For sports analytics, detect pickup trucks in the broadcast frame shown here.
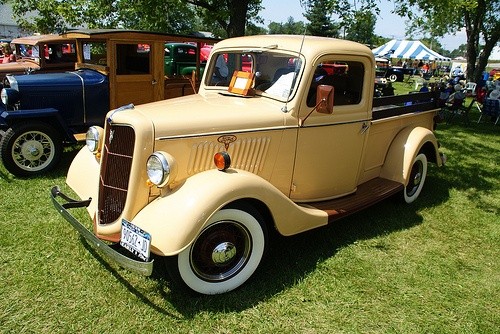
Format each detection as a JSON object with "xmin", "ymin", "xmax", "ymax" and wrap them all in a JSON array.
[
  {"xmin": 373, "ymin": 58, "xmax": 422, "ymax": 83},
  {"xmin": 51, "ymin": 32, "xmax": 445, "ymax": 291}
]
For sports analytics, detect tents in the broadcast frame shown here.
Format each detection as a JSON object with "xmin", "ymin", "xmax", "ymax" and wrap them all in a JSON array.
[
  {"xmin": 476, "ymin": 45, "xmax": 500, "ymax": 67},
  {"xmin": 371, "ymin": 40, "xmax": 451, "ymax": 64}
]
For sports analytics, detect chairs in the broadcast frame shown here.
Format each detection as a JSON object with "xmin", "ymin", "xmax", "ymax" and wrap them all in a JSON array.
[
  {"xmin": 61, "ymin": 53, "xmax": 76, "ymax": 63},
  {"xmin": 410, "ymin": 78, "xmax": 500, "ymax": 128},
  {"xmin": 320, "ymin": 74, "xmax": 349, "ymax": 108},
  {"xmin": 272, "ymin": 69, "xmax": 292, "ymax": 88}
]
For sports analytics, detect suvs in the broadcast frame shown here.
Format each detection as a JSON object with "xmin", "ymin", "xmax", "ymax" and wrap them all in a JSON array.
[
  {"xmin": 0, "ymin": 29, "xmax": 226, "ymax": 178},
  {"xmin": 1, "ymin": 33, "xmax": 93, "ymax": 85}
]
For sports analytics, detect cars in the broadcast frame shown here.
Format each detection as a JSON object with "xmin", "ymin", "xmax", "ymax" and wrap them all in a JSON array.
[{"xmin": 161, "ymin": 43, "xmax": 251, "ymax": 86}]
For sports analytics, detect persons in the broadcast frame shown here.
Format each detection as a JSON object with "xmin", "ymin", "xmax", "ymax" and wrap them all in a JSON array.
[
  {"xmin": 420, "ymin": 65, "xmax": 500, "ymax": 119},
  {"xmin": 1, "ymin": 42, "xmax": 16, "ymax": 64},
  {"xmin": 386, "ymin": 57, "xmax": 451, "ymax": 76},
  {"xmin": 307, "ymin": 66, "xmax": 329, "ymax": 106}
]
